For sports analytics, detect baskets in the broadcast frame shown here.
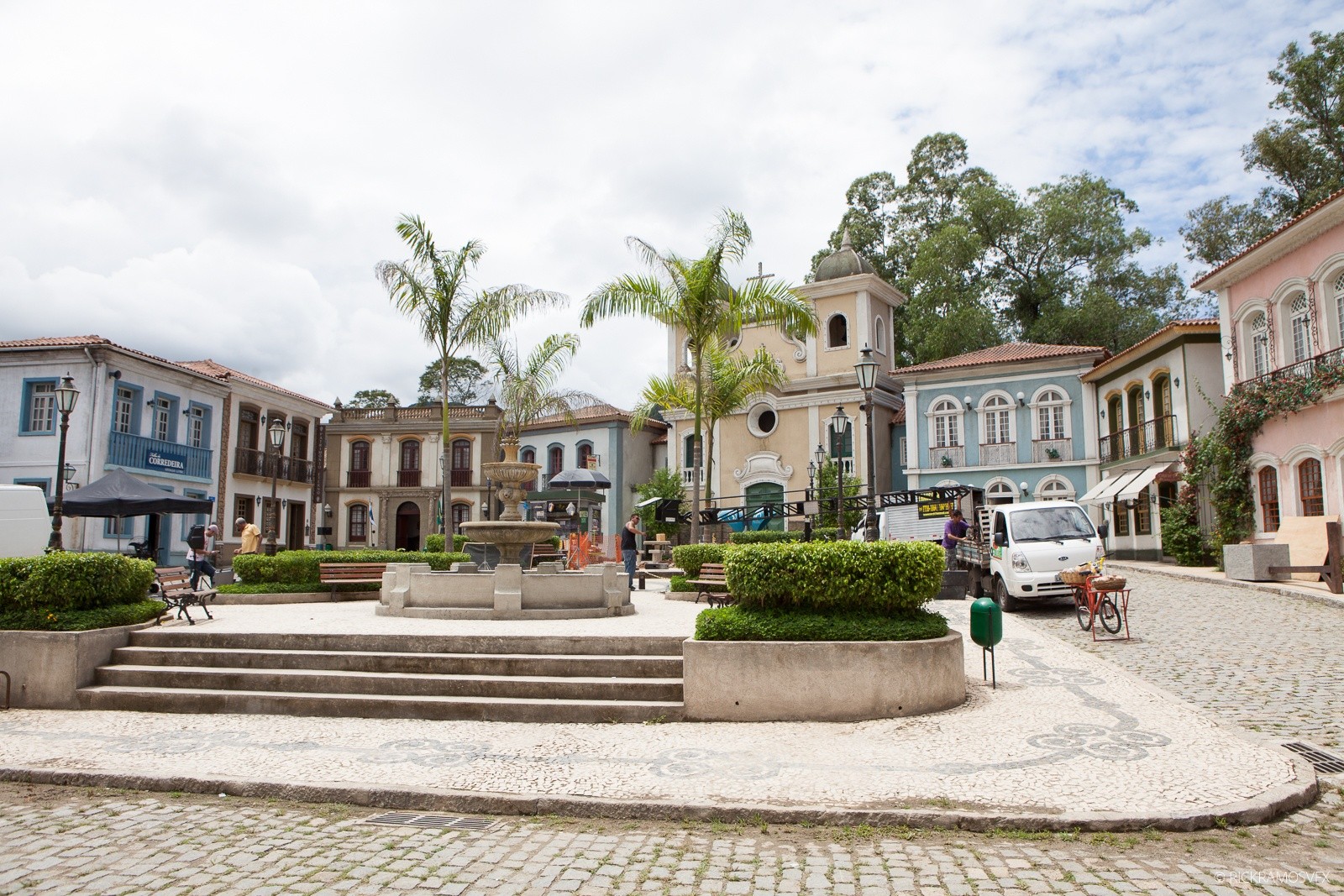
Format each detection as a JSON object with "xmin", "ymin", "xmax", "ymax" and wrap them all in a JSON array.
[
  {"xmin": 1058, "ymin": 571, "xmax": 1095, "ymax": 583},
  {"xmin": 1092, "ymin": 576, "xmax": 1127, "ymax": 590}
]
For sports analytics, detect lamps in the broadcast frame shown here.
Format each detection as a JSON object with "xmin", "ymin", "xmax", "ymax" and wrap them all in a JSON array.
[
  {"xmin": 480, "ymin": 501, "xmax": 489, "ymax": 517},
  {"xmin": 64, "ymin": 463, "xmax": 79, "ymax": 492},
  {"xmin": 1220, "ymin": 298, "xmax": 1315, "ymax": 361},
  {"xmin": 1174, "ymin": 377, "xmax": 1179, "ymax": 388},
  {"xmin": 282, "ymin": 499, "xmax": 287, "ymax": 509},
  {"xmin": 257, "ymin": 495, "xmax": 261, "ymax": 507},
  {"xmin": 1100, "ymin": 410, "xmax": 1105, "ymax": 418},
  {"xmin": 1105, "ymin": 502, "xmax": 1110, "ymax": 510},
  {"xmin": 1019, "ymin": 482, "xmax": 1028, "ymax": 497},
  {"xmin": 147, "ymin": 398, "xmax": 191, "ymax": 417},
  {"xmin": 286, "ymin": 422, "xmax": 291, "ymax": 432},
  {"xmin": 324, "ymin": 503, "xmax": 332, "ymax": 518},
  {"xmin": 1150, "ymin": 494, "xmax": 1157, "ymax": 504},
  {"xmin": 1017, "ymin": 392, "xmax": 1025, "ymax": 407},
  {"xmin": 964, "ymin": 396, "xmax": 972, "ymax": 411},
  {"xmin": 261, "ymin": 415, "xmax": 266, "ymax": 427},
  {"xmin": 1144, "ymin": 390, "xmax": 1149, "ymax": 400}
]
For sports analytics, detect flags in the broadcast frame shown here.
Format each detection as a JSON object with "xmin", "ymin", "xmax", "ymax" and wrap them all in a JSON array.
[
  {"xmin": 369, "ymin": 503, "xmax": 375, "ymax": 526},
  {"xmin": 437, "ymin": 500, "xmax": 442, "ymax": 526}
]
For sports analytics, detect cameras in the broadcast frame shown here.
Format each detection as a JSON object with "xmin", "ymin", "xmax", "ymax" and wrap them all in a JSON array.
[{"xmin": 212, "ymin": 550, "xmax": 220, "ymax": 555}]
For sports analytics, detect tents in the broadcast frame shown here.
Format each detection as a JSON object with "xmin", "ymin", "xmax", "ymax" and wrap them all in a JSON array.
[{"xmin": 45, "ymin": 467, "xmax": 213, "ymax": 555}]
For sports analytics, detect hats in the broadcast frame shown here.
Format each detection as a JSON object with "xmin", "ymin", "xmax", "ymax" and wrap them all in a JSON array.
[{"xmin": 209, "ymin": 524, "xmax": 219, "ymax": 535}]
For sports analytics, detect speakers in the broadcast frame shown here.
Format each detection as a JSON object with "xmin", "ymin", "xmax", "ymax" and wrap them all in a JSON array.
[{"xmin": 186, "ymin": 524, "xmax": 205, "ymax": 549}]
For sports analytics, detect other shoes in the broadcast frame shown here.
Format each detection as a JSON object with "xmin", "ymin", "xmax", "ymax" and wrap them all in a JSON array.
[{"xmin": 630, "ymin": 586, "xmax": 635, "ymax": 591}]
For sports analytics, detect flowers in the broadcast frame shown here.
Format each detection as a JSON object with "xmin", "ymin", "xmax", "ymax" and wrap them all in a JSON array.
[
  {"xmin": 940, "ymin": 455, "xmax": 952, "ymax": 465},
  {"xmin": 1045, "ymin": 449, "xmax": 1060, "ymax": 459}
]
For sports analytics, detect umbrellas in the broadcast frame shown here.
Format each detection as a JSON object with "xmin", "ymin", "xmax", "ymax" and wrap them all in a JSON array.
[{"xmin": 548, "ymin": 466, "xmax": 612, "ymax": 568}]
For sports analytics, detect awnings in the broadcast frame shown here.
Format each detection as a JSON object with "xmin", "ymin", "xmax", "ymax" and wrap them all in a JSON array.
[
  {"xmin": 1077, "ymin": 471, "xmax": 1128, "ymax": 506},
  {"xmin": 1118, "ymin": 463, "xmax": 1173, "ymax": 501},
  {"xmin": 1094, "ymin": 470, "xmax": 1144, "ymax": 504}
]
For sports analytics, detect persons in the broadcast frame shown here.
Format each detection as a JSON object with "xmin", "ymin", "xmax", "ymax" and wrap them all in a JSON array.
[
  {"xmin": 620, "ymin": 513, "xmax": 647, "ymax": 591},
  {"xmin": 942, "ymin": 510, "xmax": 976, "ymax": 570},
  {"xmin": 233, "ymin": 518, "xmax": 263, "ymax": 555},
  {"xmin": 186, "ymin": 525, "xmax": 218, "ymax": 592}
]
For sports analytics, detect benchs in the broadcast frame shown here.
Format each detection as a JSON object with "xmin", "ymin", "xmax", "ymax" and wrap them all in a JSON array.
[
  {"xmin": 532, "ymin": 544, "xmax": 569, "ymax": 571},
  {"xmin": 154, "ymin": 565, "xmax": 220, "ymax": 625},
  {"xmin": 685, "ymin": 563, "xmax": 737, "ymax": 608},
  {"xmin": 319, "ymin": 562, "xmax": 386, "ymax": 602}
]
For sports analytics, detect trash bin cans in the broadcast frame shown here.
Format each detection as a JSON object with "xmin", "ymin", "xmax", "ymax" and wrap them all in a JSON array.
[
  {"xmin": 326, "ymin": 543, "xmax": 332, "ymax": 551},
  {"xmin": 970, "ymin": 596, "xmax": 1003, "ymax": 648},
  {"xmin": 316, "ymin": 544, "xmax": 323, "ymax": 551}
]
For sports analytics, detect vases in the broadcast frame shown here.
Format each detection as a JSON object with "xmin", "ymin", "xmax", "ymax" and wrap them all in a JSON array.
[
  {"xmin": 941, "ymin": 464, "xmax": 953, "ymax": 468},
  {"xmin": 1049, "ymin": 458, "xmax": 1062, "ymax": 461}
]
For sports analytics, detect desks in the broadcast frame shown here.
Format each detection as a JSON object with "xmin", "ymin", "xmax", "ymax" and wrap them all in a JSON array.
[{"xmin": 643, "ymin": 540, "xmax": 671, "ymax": 562}]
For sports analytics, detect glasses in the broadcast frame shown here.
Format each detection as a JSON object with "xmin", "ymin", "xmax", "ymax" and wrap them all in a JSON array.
[{"xmin": 636, "ymin": 517, "xmax": 640, "ymax": 520}]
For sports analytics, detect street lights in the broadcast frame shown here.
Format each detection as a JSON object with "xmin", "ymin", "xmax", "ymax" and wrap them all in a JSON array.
[
  {"xmin": 482, "ymin": 476, "xmax": 493, "ymax": 520},
  {"xmin": 805, "ymin": 461, "xmax": 817, "ymax": 529},
  {"xmin": 854, "ymin": 342, "xmax": 882, "ymax": 544},
  {"xmin": 264, "ymin": 418, "xmax": 286, "ymax": 557},
  {"xmin": 814, "ymin": 443, "xmax": 826, "ymax": 526},
  {"xmin": 832, "ymin": 405, "xmax": 849, "ymax": 539},
  {"xmin": 439, "ymin": 452, "xmax": 446, "ymax": 535},
  {"xmin": 43, "ymin": 369, "xmax": 80, "ymax": 557}
]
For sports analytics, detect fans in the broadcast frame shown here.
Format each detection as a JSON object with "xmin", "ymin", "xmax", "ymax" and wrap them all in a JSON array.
[{"xmin": 126, "ymin": 536, "xmax": 151, "ymax": 559}]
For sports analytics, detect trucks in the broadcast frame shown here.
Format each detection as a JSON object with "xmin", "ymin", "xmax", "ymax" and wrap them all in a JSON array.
[
  {"xmin": 0, "ymin": 484, "xmax": 53, "ymax": 560},
  {"xmin": 956, "ymin": 501, "xmax": 1111, "ymax": 611},
  {"xmin": 851, "ymin": 484, "xmax": 986, "ymax": 549}
]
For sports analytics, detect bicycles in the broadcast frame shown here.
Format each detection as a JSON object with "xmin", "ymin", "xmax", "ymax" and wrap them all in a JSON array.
[{"xmin": 1073, "ymin": 552, "xmax": 1123, "ymax": 634}]
